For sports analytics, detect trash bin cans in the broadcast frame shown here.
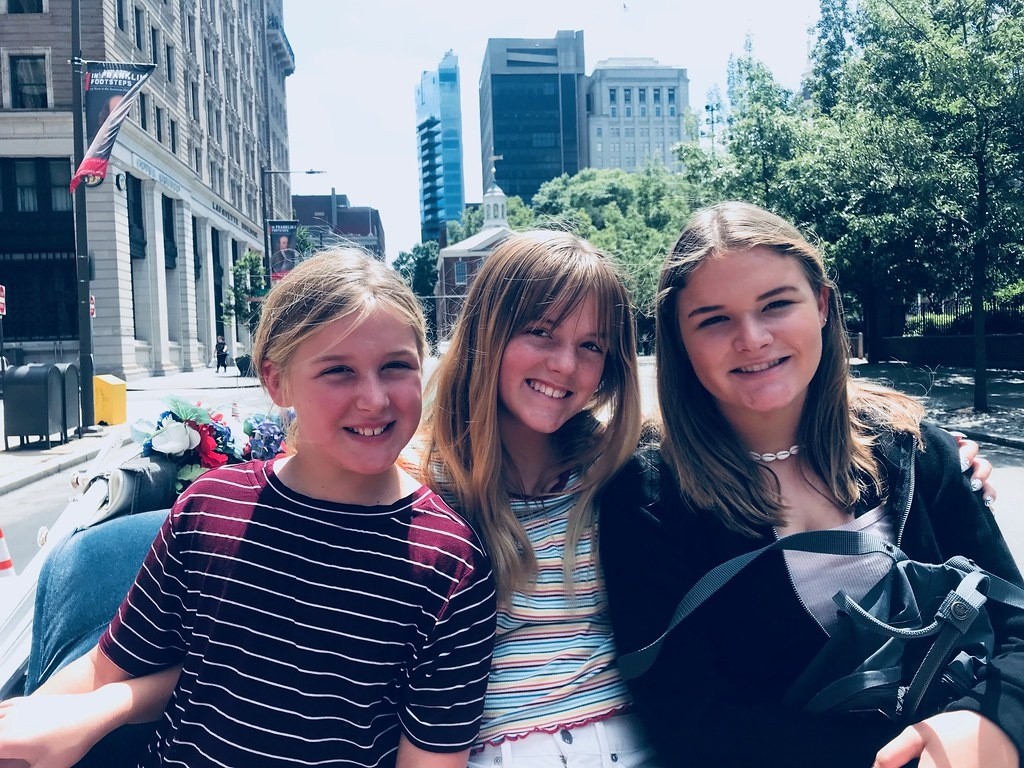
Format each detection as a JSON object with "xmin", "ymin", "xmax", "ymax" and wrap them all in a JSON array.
[
  {"xmin": 2, "ymin": 365, "xmax": 64, "ymax": 452},
  {"xmin": 643, "ymin": 341, "xmax": 655, "ymax": 356},
  {"xmin": 26, "ymin": 362, "xmax": 82, "ymax": 443},
  {"xmin": 636, "ymin": 341, "xmax": 643, "ymax": 356}
]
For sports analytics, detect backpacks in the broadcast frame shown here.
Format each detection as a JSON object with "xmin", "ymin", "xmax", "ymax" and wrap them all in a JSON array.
[{"xmin": 783, "ymin": 555, "xmax": 1024, "ymax": 768}]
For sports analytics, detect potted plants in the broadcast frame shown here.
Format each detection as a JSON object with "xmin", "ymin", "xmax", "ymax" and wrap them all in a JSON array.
[{"xmin": 219, "ymin": 248, "xmax": 270, "ymax": 379}]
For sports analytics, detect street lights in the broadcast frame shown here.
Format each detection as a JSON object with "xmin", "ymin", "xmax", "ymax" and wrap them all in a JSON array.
[
  {"xmin": 260, "ymin": 166, "xmax": 325, "ymax": 290},
  {"xmin": 699, "ymin": 102, "xmax": 722, "ymax": 162}
]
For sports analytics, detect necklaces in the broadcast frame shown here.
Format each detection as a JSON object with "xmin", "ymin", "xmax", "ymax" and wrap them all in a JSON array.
[{"xmin": 748, "ymin": 445, "xmax": 804, "ymax": 463}]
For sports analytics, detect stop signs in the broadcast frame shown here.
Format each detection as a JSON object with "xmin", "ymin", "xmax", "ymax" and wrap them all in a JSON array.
[
  {"xmin": 0, "ymin": 285, "xmax": 6, "ymax": 315},
  {"xmin": 89, "ymin": 295, "xmax": 96, "ymax": 317}
]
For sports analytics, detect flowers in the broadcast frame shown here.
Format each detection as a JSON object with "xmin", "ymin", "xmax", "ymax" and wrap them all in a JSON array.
[{"xmin": 144, "ymin": 392, "xmax": 295, "ymax": 500}]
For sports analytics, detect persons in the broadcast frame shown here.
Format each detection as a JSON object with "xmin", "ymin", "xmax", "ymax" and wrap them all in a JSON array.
[
  {"xmin": 0, "ymin": 228, "xmax": 656, "ymax": 768},
  {"xmin": 272, "ymin": 236, "xmax": 295, "ymax": 273},
  {"xmin": 598, "ymin": 201, "xmax": 1024, "ymax": 768},
  {"xmin": 213, "ymin": 335, "xmax": 228, "ymax": 373},
  {"xmin": 0, "ymin": 247, "xmax": 499, "ymax": 768}
]
